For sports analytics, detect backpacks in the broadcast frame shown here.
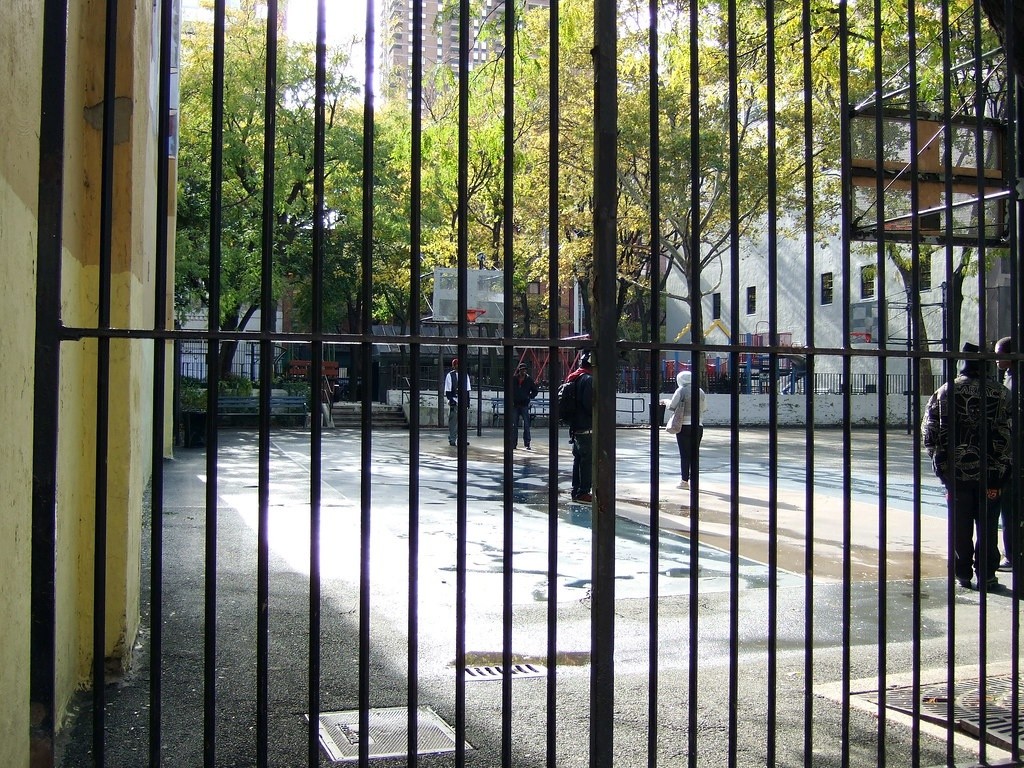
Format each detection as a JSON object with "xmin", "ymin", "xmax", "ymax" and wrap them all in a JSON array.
[{"xmin": 557, "ymin": 371, "xmax": 585, "ymax": 423}]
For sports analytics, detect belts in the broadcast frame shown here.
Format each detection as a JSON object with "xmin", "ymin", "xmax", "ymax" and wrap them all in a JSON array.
[{"xmin": 573, "ymin": 430, "xmax": 592, "ymax": 434}]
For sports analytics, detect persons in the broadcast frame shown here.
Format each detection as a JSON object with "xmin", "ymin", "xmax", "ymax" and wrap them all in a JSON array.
[
  {"xmin": 659, "ymin": 371, "xmax": 706, "ymax": 490},
  {"xmin": 512, "ymin": 363, "xmax": 538, "ymax": 451},
  {"xmin": 445, "ymin": 359, "xmax": 471, "ymax": 446},
  {"xmin": 920, "ymin": 342, "xmax": 1012, "ymax": 593},
  {"xmin": 990, "ymin": 337, "xmax": 1012, "ymax": 571},
  {"xmin": 564, "ymin": 354, "xmax": 593, "ymax": 504}
]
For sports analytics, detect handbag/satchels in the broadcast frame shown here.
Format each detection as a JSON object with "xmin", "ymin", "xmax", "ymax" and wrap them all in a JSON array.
[{"xmin": 665, "ymin": 388, "xmax": 685, "ymax": 433}]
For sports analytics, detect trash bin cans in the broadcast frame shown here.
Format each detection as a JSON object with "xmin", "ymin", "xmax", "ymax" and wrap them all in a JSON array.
[{"xmin": 649, "ymin": 403, "xmax": 666, "ymax": 426}]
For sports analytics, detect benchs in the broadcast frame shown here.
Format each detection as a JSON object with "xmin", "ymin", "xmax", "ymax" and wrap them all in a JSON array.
[
  {"xmin": 215, "ymin": 395, "xmax": 309, "ymax": 430},
  {"xmin": 490, "ymin": 398, "xmax": 549, "ymax": 428}
]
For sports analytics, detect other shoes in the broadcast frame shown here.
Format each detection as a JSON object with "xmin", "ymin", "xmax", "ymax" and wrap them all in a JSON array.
[
  {"xmin": 450, "ymin": 441, "xmax": 456, "ymax": 446},
  {"xmin": 977, "ymin": 575, "xmax": 998, "ymax": 589},
  {"xmin": 571, "ymin": 493, "xmax": 578, "ymax": 501},
  {"xmin": 955, "ymin": 572, "xmax": 972, "ymax": 586},
  {"xmin": 467, "ymin": 442, "xmax": 469, "ymax": 445},
  {"xmin": 577, "ymin": 493, "xmax": 593, "ymax": 503},
  {"xmin": 513, "ymin": 445, "xmax": 516, "ymax": 449},
  {"xmin": 999, "ymin": 556, "xmax": 1013, "ymax": 572},
  {"xmin": 526, "ymin": 446, "xmax": 531, "ymax": 450}
]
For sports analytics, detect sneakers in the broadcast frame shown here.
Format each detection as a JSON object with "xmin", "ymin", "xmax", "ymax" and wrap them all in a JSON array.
[{"xmin": 677, "ymin": 481, "xmax": 689, "ymax": 489}]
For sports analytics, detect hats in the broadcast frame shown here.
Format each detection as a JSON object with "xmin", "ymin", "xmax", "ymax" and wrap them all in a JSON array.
[
  {"xmin": 579, "ymin": 351, "xmax": 591, "ymax": 363},
  {"xmin": 518, "ymin": 363, "xmax": 528, "ymax": 370},
  {"xmin": 452, "ymin": 359, "xmax": 458, "ymax": 366}
]
